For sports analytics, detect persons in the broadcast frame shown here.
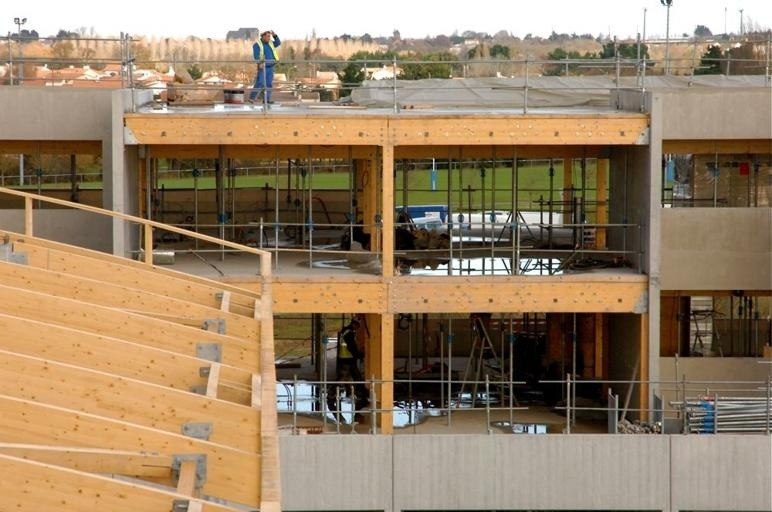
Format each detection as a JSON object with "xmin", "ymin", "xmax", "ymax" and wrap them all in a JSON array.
[
  {"xmin": 335, "ymin": 316, "xmax": 370, "ymax": 400},
  {"xmin": 247, "ymin": 30, "xmax": 281, "ymax": 105}
]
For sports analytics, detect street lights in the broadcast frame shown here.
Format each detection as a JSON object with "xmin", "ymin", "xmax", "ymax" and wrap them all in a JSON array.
[
  {"xmin": 659, "ymin": 0, "xmax": 673, "ymax": 78},
  {"xmin": 13, "ymin": 17, "xmax": 29, "ymax": 187}
]
